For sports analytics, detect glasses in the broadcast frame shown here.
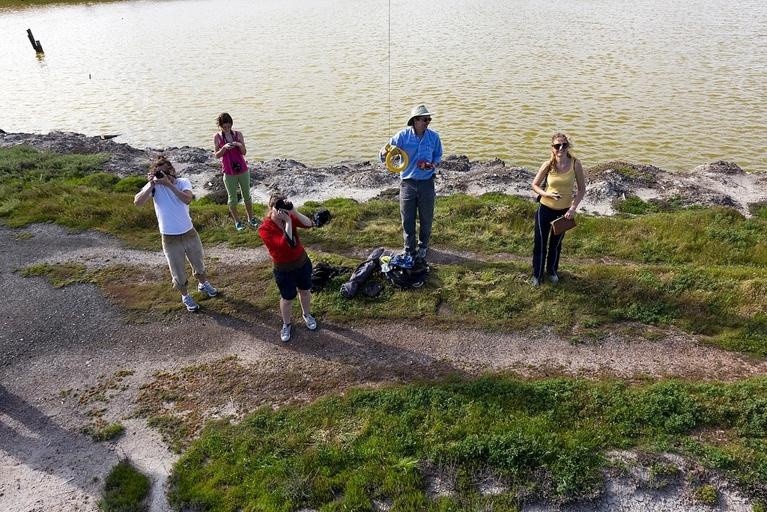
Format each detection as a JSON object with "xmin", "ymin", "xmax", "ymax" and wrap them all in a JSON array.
[
  {"xmin": 551, "ymin": 143, "xmax": 570, "ymax": 150},
  {"xmin": 422, "ymin": 118, "xmax": 432, "ymax": 122}
]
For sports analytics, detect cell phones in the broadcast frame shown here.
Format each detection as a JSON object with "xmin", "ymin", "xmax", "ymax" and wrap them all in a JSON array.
[
  {"xmin": 229, "ymin": 143, "xmax": 234, "ymax": 147},
  {"xmin": 554, "ymin": 193, "xmax": 561, "ymax": 200}
]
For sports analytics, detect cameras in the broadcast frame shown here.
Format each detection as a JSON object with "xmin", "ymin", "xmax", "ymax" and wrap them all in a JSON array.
[
  {"xmin": 232, "ymin": 162, "xmax": 243, "ymax": 173},
  {"xmin": 153, "ymin": 167, "xmax": 170, "ymax": 181},
  {"xmin": 274, "ymin": 198, "xmax": 294, "ymax": 217}
]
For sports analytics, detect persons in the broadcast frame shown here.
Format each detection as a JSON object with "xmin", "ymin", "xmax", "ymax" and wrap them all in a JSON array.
[
  {"xmin": 213, "ymin": 110, "xmax": 257, "ymax": 231},
  {"xmin": 530, "ymin": 132, "xmax": 586, "ymax": 283},
  {"xmin": 378, "ymin": 103, "xmax": 445, "ymax": 264},
  {"xmin": 133, "ymin": 157, "xmax": 218, "ymax": 312},
  {"xmin": 256, "ymin": 185, "xmax": 320, "ymax": 342}
]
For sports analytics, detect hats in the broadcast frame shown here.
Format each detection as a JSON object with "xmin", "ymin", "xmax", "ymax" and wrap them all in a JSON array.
[{"xmin": 407, "ymin": 104, "xmax": 435, "ymax": 126}]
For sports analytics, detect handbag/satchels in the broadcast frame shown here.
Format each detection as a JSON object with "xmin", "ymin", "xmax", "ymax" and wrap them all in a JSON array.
[
  {"xmin": 315, "ymin": 210, "xmax": 331, "ymax": 227},
  {"xmin": 531, "ymin": 176, "xmax": 547, "ymax": 203},
  {"xmin": 310, "ymin": 261, "xmax": 348, "ymax": 291}
]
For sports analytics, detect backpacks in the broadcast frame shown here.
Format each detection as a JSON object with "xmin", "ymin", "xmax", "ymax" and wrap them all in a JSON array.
[{"xmin": 384, "ymin": 254, "xmax": 432, "ymax": 289}]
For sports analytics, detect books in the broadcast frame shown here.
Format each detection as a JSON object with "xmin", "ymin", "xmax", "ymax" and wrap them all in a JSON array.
[{"xmin": 550, "ymin": 215, "xmax": 576, "ymax": 235}]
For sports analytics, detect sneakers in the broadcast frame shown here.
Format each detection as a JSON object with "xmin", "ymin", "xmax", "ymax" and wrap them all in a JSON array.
[
  {"xmin": 417, "ymin": 247, "xmax": 429, "ymax": 258},
  {"xmin": 280, "ymin": 323, "xmax": 292, "ymax": 342},
  {"xmin": 198, "ymin": 281, "xmax": 219, "ymax": 297},
  {"xmin": 182, "ymin": 293, "xmax": 200, "ymax": 312},
  {"xmin": 533, "ymin": 276, "xmax": 541, "ymax": 286},
  {"xmin": 248, "ymin": 217, "xmax": 263, "ymax": 228},
  {"xmin": 302, "ymin": 314, "xmax": 317, "ymax": 329},
  {"xmin": 234, "ymin": 221, "xmax": 246, "ymax": 231},
  {"xmin": 549, "ymin": 275, "xmax": 558, "ymax": 283}
]
